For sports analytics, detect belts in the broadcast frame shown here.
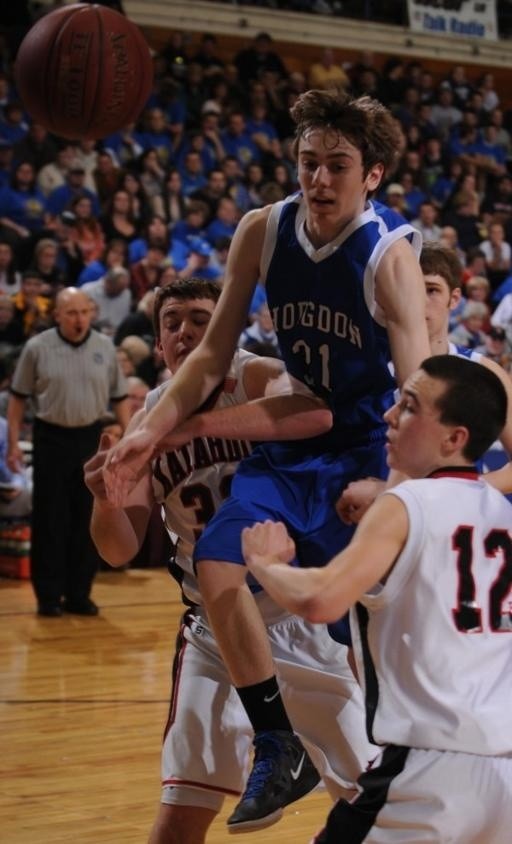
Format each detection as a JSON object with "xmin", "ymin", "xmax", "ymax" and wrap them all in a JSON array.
[{"xmin": 35, "ymin": 417, "xmax": 104, "ymax": 435}]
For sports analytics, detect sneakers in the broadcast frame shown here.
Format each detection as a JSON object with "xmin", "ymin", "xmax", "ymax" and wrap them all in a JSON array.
[{"xmin": 226, "ymin": 729, "xmax": 325, "ymax": 838}]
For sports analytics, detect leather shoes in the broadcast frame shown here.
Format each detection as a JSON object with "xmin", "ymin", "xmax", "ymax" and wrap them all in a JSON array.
[
  {"xmin": 36, "ymin": 598, "xmax": 65, "ymax": 618},
  {"xmin": 63, "ymin": 594, "xmax": 99, "ymax": 617}
]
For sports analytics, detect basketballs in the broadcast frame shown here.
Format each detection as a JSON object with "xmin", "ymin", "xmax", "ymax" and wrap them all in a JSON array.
[{"xmin": 14, "ymin": 5, "xmax": 152, "ymax": 135}]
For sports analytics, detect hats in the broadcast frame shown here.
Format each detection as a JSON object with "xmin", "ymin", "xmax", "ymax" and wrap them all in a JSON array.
[
  {"xmin": 189, "ymin": 238, "xmax": 212, "ymax": 258},
  {"xmin": 30, "ymin": 231, "xmax": 61, "ymax": 255}
]
